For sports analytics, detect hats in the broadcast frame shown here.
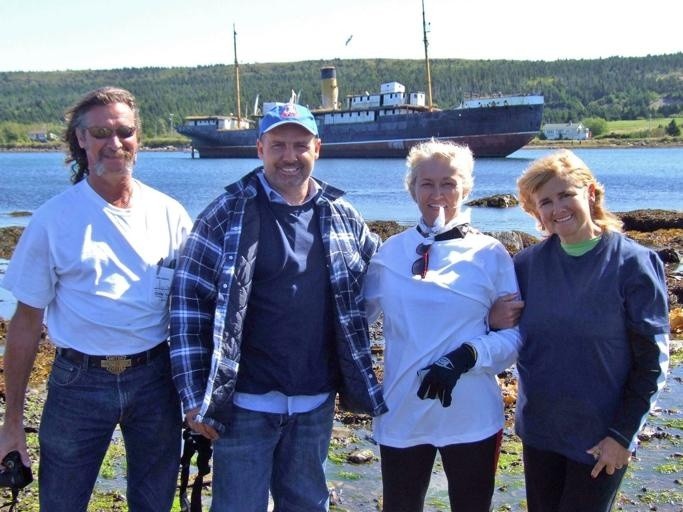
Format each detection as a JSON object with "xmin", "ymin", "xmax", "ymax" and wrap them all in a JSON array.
[{"xmin": 257, "ymin": 103, "xmax": 319, "ymax": 140}]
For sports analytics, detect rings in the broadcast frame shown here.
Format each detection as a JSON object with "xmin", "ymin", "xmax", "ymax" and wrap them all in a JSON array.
[{"xmin": 616, "ymin": 466, "xmax": 623, "ymax": 470}]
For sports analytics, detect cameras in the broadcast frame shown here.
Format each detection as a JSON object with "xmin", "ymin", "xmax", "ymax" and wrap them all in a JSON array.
[{"xmin": 0, "ymin": 451, "xmax": 33, "ymax": 489}]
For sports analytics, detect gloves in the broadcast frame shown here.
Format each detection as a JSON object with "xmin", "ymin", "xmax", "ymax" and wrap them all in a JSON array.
[{"xmin": 417, "ymin": 343, "xmax": 476, "ymax": 408}]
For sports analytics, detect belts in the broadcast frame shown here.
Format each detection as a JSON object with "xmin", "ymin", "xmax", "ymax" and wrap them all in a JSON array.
[{"xmin": 55, "ymin": 338, "xmax": 168, "ymax": 376}]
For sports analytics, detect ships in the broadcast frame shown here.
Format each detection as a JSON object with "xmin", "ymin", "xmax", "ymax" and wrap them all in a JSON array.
[{"xmin": 171, "ymin": 0, "xmax": 547, "ymax": 161}]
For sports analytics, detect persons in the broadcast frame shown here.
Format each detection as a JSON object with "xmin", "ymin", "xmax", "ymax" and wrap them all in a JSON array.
[
  {"xmin": 0, "ymin": 87, "xmax": 194, "ymax": 512},
  {"xmin": 169, "ymin": 104, "xmax": 389, "ymax": 512},
  {"xmin": 489, "ymin": 149, "xmax": 670, "ymax": 512},
  {"xmin": 364, "ymin": 136, "xmax": 523, "ymax": 512}
]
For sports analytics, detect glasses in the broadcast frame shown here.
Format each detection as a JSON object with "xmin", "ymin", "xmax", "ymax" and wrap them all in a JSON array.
[
  {"xmin": 85, "ymin": 127, "xmax": 138, "ymax": 138},
  {"xmin": 412, "ymin": 240, "xmax": 433, "ymax": 279}
]
